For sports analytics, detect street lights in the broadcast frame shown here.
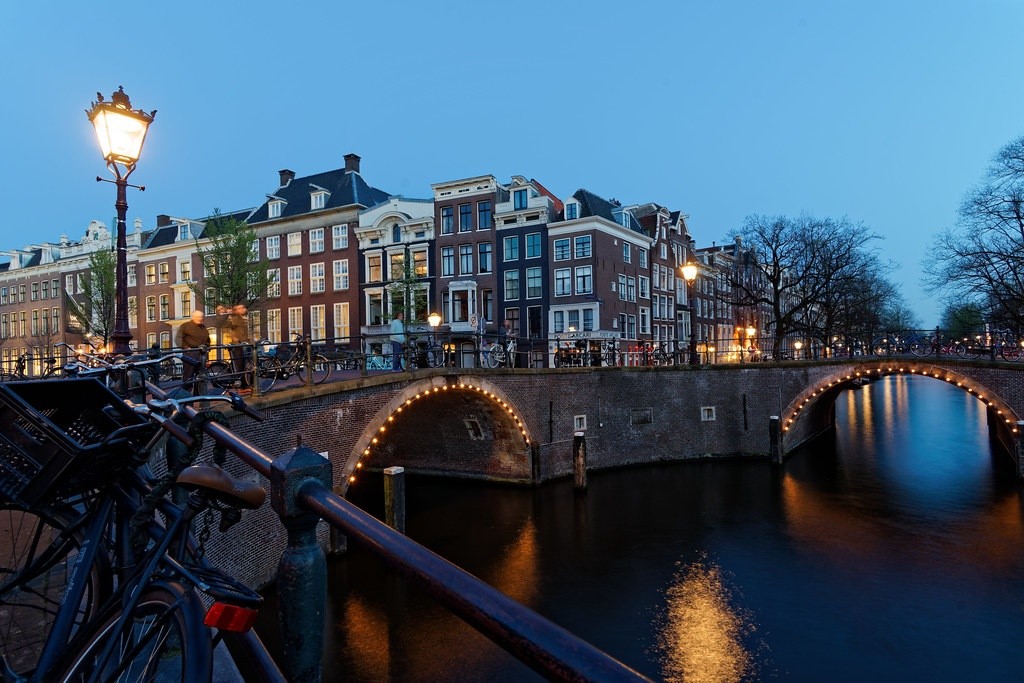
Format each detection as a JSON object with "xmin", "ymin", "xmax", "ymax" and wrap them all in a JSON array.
[
  {"xmin": 746, "ymin": 326, "xmax": 756, "ymax": 349},
  {"xmin": 84, "ymin": 84, "xmax": 158, "ymax": 356},
  {"xmin": 794, "ymin": 341, "xmax": 803, "ymax": 360},
  {"xmin": 679, "ymin": 260, "xmax": 701, "ymax": 366}
]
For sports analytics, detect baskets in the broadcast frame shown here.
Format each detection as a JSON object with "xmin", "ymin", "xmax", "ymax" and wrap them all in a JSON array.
[{"xmin": 0, "ymin": 377, "xmax": 151, "ymax": 502}]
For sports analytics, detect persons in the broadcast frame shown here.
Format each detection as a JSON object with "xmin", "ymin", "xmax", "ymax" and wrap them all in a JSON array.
[
  {"xmin": 498, "ymin": 320, "xmax": 512, "ymax": 367},
  {"xmin": 390, "ymin": 312, "xmax": 406, "ymax": 372},
  {"xmin": 174, "ymin": 311, "xmax": 211, "ymax": 396}
]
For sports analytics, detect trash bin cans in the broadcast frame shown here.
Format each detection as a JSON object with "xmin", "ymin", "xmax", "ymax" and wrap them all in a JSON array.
[{"xmin": 224, "ymin": 341, "xmax": 290, "ymax": 388}]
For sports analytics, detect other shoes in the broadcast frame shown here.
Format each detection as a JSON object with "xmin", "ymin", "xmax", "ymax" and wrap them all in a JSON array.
[
  {"xmin": 392, "ymin": 369, "xmax": 402, "ymax": 372},
  {"xmin": 239, "ymin": 389, "xmax": 253, "ymax": 395}
]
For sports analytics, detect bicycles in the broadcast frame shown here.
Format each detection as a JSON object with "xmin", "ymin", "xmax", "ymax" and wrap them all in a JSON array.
[
  {"xmin": 487, "ymin": 332, "xmax": 518, "ymax": 369},
  {"xmin": 554, "ymin": 339, "xmax": 623, "ymax": 368},
  {"xmin": 650, "ymin": 339, "xmax": 689, "ymax": 367},
  {"xmin": 956, "ymin": 328, "xmax": 1023, "ymax": 363},
  {"xmin": 871, "ymin": 327, "xmax": 934, "ymax": 358},
  {"xmin": 398, "ymin": 325, "xmax": 447, "ymax": 373},
  {"xmin": 0, "ymin": 333, "xmax": 396, "ymax": 683}
]
